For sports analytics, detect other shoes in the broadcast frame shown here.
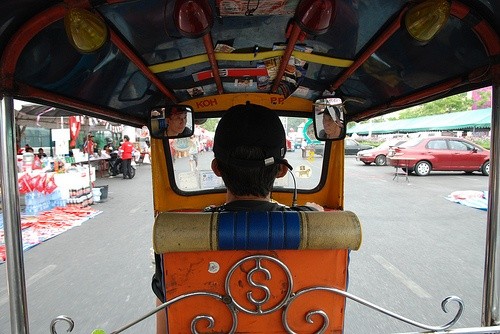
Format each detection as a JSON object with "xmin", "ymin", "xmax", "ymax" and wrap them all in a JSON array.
[{"xmin": 109, "ymin": 175, "xmax": 116, "ymax": 178}]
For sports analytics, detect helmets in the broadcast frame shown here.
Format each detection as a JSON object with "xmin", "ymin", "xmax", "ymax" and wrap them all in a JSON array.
[{"xmin": 106, "ymin": 137, "xmax": 113, "ymax": 143}]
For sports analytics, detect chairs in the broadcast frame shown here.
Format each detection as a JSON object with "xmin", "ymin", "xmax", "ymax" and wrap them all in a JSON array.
[{"xmin": 153, "ymin": 209, "xmax": 362, "ymax": 334}]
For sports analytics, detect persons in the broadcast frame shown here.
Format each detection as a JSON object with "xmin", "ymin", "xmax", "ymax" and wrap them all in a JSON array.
[
  {"xmin": 16, "ymin": 135, "xmax": 137, "ymax": 180},
  {"xmin": 318, "ymin": 105, "xmax": 345, "ymax": 139},
  {"xmin": 152, "ymin": 105, "xmax": 192, "ymax": 138},
  {"xmin": 150, "ymin": 101, "xmax": 320, "ymax": 304}
]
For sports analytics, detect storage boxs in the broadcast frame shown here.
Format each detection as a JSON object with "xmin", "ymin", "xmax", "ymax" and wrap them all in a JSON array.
[{"xmin": 95, "ymin": 185, "xmax": 109, "ymax": 200}]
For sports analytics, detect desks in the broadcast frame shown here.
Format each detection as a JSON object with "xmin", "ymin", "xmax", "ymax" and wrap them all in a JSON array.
[
  {"xmin": 76, "ymin": 157, "xmax": 111, "ymax": 180},
  {"xmin": 389, "ymin": 157, "xmax": 416, "ymax": 181}
]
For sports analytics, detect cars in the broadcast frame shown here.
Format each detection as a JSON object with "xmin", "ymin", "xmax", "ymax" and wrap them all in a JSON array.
[
  {"xmin": 386, "ymin": 136, "xmax": 490, "ymax": 177},
  {"xmin": 357, "ymin": 139, "xmax": 407, "ymax": 166},
  {"xmin": 311, "ymin": 138, "xmax": 376, "ymax": 155}
]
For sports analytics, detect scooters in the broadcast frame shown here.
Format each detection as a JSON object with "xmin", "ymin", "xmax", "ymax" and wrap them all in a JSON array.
[{"xmin": 107, "ymin": 159, "xmax": 136, "ymax": 179}]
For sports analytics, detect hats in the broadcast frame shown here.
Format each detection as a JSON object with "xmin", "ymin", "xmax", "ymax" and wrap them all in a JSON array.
[
  {"xmin": 165, "ymin": 107, "xmax": 191, "ymax": 117},
  {"xmin": 317, "ymin": 105, "xmax": 340, "ymax": 119},
  {"xmin": 213, "ymin": 101, "xmax": 294, "ymax": 170}
]
